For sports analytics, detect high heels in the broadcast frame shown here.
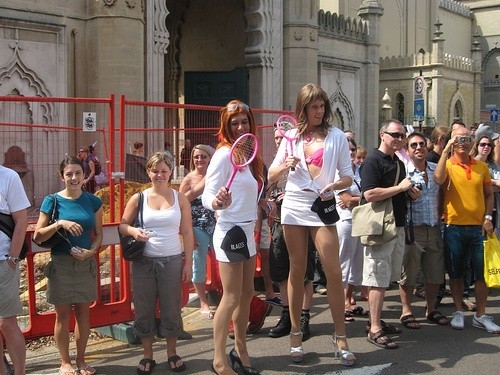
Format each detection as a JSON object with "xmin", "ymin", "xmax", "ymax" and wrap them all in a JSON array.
[
  {"xmin": 210, "ymin": 359, "xmax": 241, "ymax": 375},
  {"xmin": 229, "ymin": 347, "xmax": 260, "ymax": 375},
  {"xmin": 289, "ymin": 329, "xmax": 304, "ymax": 363},
  {"xmin": 329, "ymin": 331, "xmax": 356, "ymax": 366}
]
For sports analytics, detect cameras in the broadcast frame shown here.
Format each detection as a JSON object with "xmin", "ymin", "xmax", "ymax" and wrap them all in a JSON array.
[
  {"xmin": 406, "ymin": 183, "xmax": 423, "ymax": 201},
  {"xmin": 457, "ymin": 136, "xmax": 472, "ymax": 146},
  {"xmin": 142, "ymin": 230, "xmax": 158, "ymax": 237}
]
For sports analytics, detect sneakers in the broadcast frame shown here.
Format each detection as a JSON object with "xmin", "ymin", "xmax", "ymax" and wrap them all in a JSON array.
[
  {"xmin": 472, "ymin": 312, "xmax": 500, "ymax": 334},
  {"xmin": 450, "ymin": 311, "xmax": 465, "ymax": 330},
  {"xmin": 462, "ymin": 296, "xmax": 476, "ymax": 311},
  {"xmin": 416, "ymin": 287, "xmax": 425, "ymax": 297},
  {"xmin": 316, "ymin": 284, "xmax": 325, "ymax": 294},
  {"xmin": 248, "ymin": 300, "xmax": 273, "ymax": 334}
]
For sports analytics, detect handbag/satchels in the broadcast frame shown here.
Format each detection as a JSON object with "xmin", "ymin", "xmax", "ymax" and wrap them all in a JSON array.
[
  {"xmin": 32, "ymin": 218, "xmax": 73, "ymax": 249},
  {"xmin": 122, "ymin": 223, "xmax": 146, "ymax": 260},
  {"xmin": 93, "ymin": 162, "xmax": 101, "ymax": 175},
  {"xmin": 482, "ymin": 233, "xmax": 500, "ymax": 289},
  {"xmin": 0, "ymin": 212, "xmax": 28, "ymax": 259},
  {"xmin": 350, "ymin": 196, "xmax": 398, "ymax": 247}
]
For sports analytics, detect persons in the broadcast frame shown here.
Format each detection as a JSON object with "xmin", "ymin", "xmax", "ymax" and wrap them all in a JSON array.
[
  {"xmin": 164, "ymin": 141, "xmax": 177, "ymax": 170},
  {"xmin": 179, "ymin": 143, "xmax": 216, "ymax": 320},
  {"xmin": 268, "ymin": 122, "xmax": 316, "ymax": 342},
  {"xmin": 344, "ymin": 131, "xmax": 353, "ymax": 138},
  {"xmin": 396, "ymin": 133, "xmax": 449, "ymax": 329},
  {"xmin": 1, "ymin": 163, "xmax": 31, "ymax": 375},
  {"xmin": 425, "ymin": 132, "xmax": 479, "ymax": 311},
  {"xmin": 470, "ymin": 123, "xmax": 480, "ymax": 136},
  {"xmin": 466, "ymin": 135, "xmax": 500, "ymax": 286},
  {"xmin": 89, "ymin": 145, "xmax": 98, "ymax": 188},
  {"xmin": 312, "ymin": 251, "xmax": 327, "ymax": 296},
  {"xmin": 267, "ymin": 83, "xmax": 356, "ymax": 366},
  {"xmin": 468, "ymin": 125, "xmax": 500, "ymax": 168},
  {"xmin": 451, "ymin": 121, "xmax": 465, "ymax": 133},
  {"xmin": 76, "ymin": 146, "xmax": 95, "ymax": 193},
  {"xmin": 132, "ymin": 143, "xmax": 144, "ymax": 157},
  {"xmin": 434, "ymin": 127, "xmax": 500, "ymax": 335},
  {"xmin": 180, "ymin": 139, "xmax": 193, "ymax": 170},
  {"xmin": 359, "ymin": 119, "xmax": 422, "ymax": 349},
  {"xmin": 118, "ymin": 152, "xmax": 193, "ymax": 375},
  {"xmin": 416, "ymin": 126, "xmax": 451, "ymax": 297},
  {"xmin": 350, "ymin": 144, "xmax": 369, "ymax": 305},
  {"xmin": 258, "ymin": 162, "xmax": 282, "ymax": 306},
  {"xmin": 333, "ymin": 136, "xmax": 372, "ymax": 322},
  {"xmin": 33, "ymin": 156, "xmax": 104, "ymax": 375},
  {"xmin": 155, "ymin": 224, "xmax": 198, "ymax": 340},
  {"xmin": 207, "ymin": 208, "xmax": 273, "ymax": 339},
  {"xmin": 201, "ymin": 100, "xmax": 277, "ymax": 375}
]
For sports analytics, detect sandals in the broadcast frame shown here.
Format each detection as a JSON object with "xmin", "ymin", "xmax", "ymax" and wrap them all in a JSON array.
[
  {"xmin": 344, "ymin": 306, "xmax": 368, "ymax": 317},
  {"xmin": 367, "ymin": 326, "xmax": 398, "ymax": 349},
  {"xmin": 366, "ymin": 320, "xmax": 401, "ymax": 335},
  {"xmin": 200, "ymin": 309, "xmax": 213, "ymax": 320},
  {"xmin": 344, "ymin": 307, "xmax": 355, "ymax": 321}
]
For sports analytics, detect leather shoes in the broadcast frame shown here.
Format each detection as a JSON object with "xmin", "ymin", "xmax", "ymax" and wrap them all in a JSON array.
[
  {"xmin": 268, "ymin": 312, "xmax": 291, "ymax": 338},
  {"xmin": 300, "ymin": 314, "xmax": 311, "ymax": 342}
]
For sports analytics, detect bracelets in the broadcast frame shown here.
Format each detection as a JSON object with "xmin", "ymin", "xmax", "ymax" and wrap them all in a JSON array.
[{"xmin": 485, "ymin": 214, "xmax": 493, "ymax": 221}]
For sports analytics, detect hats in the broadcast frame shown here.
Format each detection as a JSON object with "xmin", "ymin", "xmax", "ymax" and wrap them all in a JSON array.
[
  {"xmin": 221, "ymin": 225, "xmax": 251, "ymax": 264},
  {"xmin": 310, "ymin": 195, "xmax": 340, "ymax": 224},
  {"xmin": 274, "ymin": 122, "xmax": 294, "ymax": 132},
  {"xmin": 474, "ymin": 125, "xmax": 499, "ymax": 140}
]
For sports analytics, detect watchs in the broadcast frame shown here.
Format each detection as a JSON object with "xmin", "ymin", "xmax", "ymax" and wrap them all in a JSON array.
[{"xmin": 7, "ymin": 256, "xmax": 19, "ymax": 264}]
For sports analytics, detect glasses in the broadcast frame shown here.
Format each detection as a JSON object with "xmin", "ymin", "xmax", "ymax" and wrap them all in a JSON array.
[
  {"xmin": 274, "ymin": 121, "xmax": 288, "ymax": 128},
  {"xmin": 350, "ymin": 147, "xmax": 356, "ymax": 150},
  {"xmin": 479, "ymin": 142, "xmax": 492, "ymax": 146},
  {"xmin": 79, "ymin": 151, "xmax": 85, "ymax": 153},
  {"xmin": 410, "ymin": 142, "xmax": 426, "ymax": 149},
  {"xmin": 383, "ymin": 131, "xmax": 406, "ymax": 140},
  {"xmin": 227, "ymin": 103, "xmax": 251, "ymax": 112},
  {"xmin": 192, "ymin": 154, "xmax": 209, "ymax": 159}
]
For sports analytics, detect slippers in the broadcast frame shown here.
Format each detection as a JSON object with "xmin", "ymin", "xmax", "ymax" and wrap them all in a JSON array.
[
  {"xmin": 424, "ymin": 310, "xmax": 449, "ymax": 326},
  {"xmin": 264, "ymin": 296, "xmax": 284, "ymax": 306},
  {"xmin": 168, "ymin": 355, "xmax": 185, "ymax": 372},
  {"xmin": 136, "ymin": 359, "xmax": 156, "ymax": 375},
  {"xmin": 72, "ymin": 365, "xmax": 96, "ymax": 375},
  {"xmin": 399, "ymin": 313, "xmax": 422, "ymax": 329},
  {"xmin": 59, "ymin": 367, "xmax": 76, "ymax": 375}
]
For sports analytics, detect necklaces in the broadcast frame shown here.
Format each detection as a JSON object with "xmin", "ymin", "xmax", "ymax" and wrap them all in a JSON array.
[
  {"xmin": 416, "ymin": 167, "xmax": 427, "ymax": 177},
  {"xmin": 305, "ymin": 128, "xmax": 314, "ymax": 142}
]
